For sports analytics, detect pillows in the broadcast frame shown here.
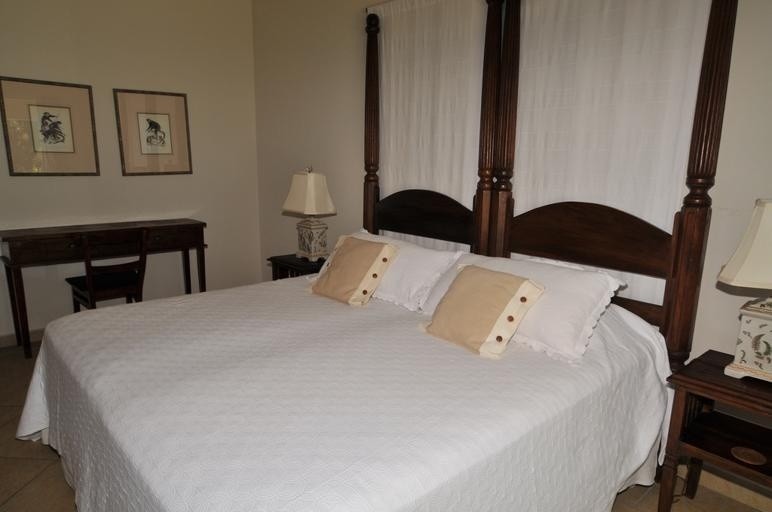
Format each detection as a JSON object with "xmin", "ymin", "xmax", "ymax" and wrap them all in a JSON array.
[
  {"xmin": 309, "ymin": 235, "xmax": 396, "ymax": 309},
  {"xmin": 424, "ymin": 264, "xmax": 544, "ymax": 358},
  {"xmin": 421, "ymin": 251, "xmax": 626, "ymax": 361},
  {"xmin": 348, "ymin": 230, "xmax": 463, "ymax": 311}
]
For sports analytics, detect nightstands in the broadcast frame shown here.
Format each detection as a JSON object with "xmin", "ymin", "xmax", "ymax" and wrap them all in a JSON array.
[
  {"xmin": 267, "ymin": 254, "xmax": 326, "ymax": 281},
  {"xmin": 658, "ymin": 350, "xmax": 772, "ymax": 512}
]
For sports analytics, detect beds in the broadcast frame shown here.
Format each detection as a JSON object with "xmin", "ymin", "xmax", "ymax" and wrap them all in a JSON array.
[{"xmin": 42, "ymin": 0, "xmax": 739, "ymax": 512}]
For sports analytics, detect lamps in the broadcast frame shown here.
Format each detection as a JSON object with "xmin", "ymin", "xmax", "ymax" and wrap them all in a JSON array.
[
  {"xmin": 282, "ymin": 164, "xmax": 337, "ymax": 261},
  {"xmin": 717, "ymin": 200, "xmax": 772, "ymax": 384}
]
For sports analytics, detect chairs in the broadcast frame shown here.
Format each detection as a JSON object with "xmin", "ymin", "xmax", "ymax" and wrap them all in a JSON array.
[{"xmin": 66, "ymin": 229, "xmax": 149, "ymax": 313}]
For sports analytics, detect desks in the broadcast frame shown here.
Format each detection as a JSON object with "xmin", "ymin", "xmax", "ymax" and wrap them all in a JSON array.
[{"xmin": 0, "ymin": 218, "xmax": 208, "ymax": 359}]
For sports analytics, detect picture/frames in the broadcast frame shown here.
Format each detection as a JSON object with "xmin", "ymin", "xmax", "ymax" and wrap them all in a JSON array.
[
  {"xmin": 113, "ymin": 87, "xmax": 194, "ymax": 176},
  {"xmin": 0, "ymin": 75, "xmax": 101, "ymax": 176}
]
